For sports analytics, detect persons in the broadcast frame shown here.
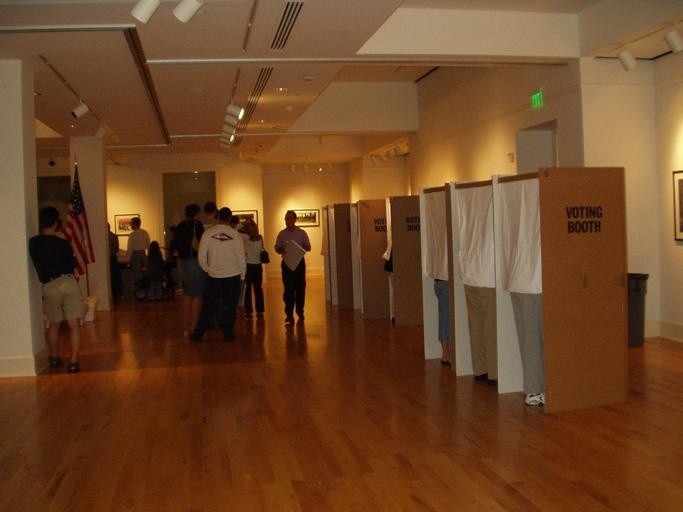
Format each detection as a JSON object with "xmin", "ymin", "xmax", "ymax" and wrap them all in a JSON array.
[
  {"xmin": 169, "ymin": 204, "xmax": 201, "ymax": 335},
  {"xmin": 460, "ymin": 282, "xmax": 500, "ymax": 385},
  {"xmin": 165, "ymin": 198, "xmax": 268, "ymax": 318},
  {"xmin": 433, "ymin": 280, "xmax": 448, "ymax": 364},
  {"xmin": 147, "ymin": 241, "xmax": 165, "ymax": 298},
  {"xmin": 125, "ymin": 217, "xmax": 150, "ymax": 297},
  {"xmin": 106, "ymin": 223, "xmax": 120, "ymax": 304},
  {"xmin": 383, "ymin": 252, "xmax": 393, "ymax": 323},
  {"xmin": 274, "ymin": 210, "xmax": 311, "ymax": 322},
  {"xmin": 515, "ymin": 292, "xmax": 546, "ymax": 407},
  {"xmin": 188, "ymin": 208, "xmax": 246, "ymax": 342},
  {"xmin": 28, "ymin": 206, "xmax": 84, "ymax": 372}
]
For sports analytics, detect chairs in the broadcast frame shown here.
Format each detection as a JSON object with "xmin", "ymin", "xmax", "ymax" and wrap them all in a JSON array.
[{"xmin": 116, "ymin": 248, "xmax": 178, "ymax": 306}]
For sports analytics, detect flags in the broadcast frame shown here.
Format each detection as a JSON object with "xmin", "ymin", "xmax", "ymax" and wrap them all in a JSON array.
[{"xmin": 65, "ymin": 166, "xmax": 96, "ymax": 279}]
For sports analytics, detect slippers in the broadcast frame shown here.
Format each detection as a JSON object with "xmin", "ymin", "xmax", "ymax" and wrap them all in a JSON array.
[{"xmin": 441, "ymin": 360, "xmax": 449, "ymax": 365}]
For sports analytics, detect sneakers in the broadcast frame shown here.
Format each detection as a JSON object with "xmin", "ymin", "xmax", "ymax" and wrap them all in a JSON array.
[
  {"xmin": 474, "ymin": 373, "xmax": 488, "ymax": 382},
  {"xmin": 183, "ymin": 329, "xmax": 190, "ymax": 338},
  {"xmin": 524, "ymin": 393, "xmax": 545, "ymax": 406},
  {"xmin": 256, "ymin": 312, "xmax": 264, "ymax": 317},
  {"xmin": 68, "ymin": 362, "xmax": 80, "ymax": 372},
  {"xmin": 246, "ymin": 313, "xmax": 253, "ymax": 318},
  {"xmin": 285, "ymin": 316, "xmax": 294, "ymax": 323},
  {"xmin": 487, "ymin": 379, "xmax": 497, "ymax": 386},
  {"xmin": 50, "ymin": 357, "xmax": 64, "ymax": 368},
  {"xmin": 298, "ymin": 314, "xmax": 305, "ymax": 321}
]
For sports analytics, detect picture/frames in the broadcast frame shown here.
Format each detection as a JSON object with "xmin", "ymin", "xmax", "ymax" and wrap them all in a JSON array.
[
  {"xmin": 286, "ymin": 208, "xmax": 321, "ymax": 228},
  {"xmin": 112, "ymin": 213, "xmax": 139, "ymax": 238},
  {"xmin": 670, "ymin": 168, "xmax": 682, "ymax": 244},
  {"xmin": 231, "ymin": 209, "xmax": 257, "ymax": 232}
]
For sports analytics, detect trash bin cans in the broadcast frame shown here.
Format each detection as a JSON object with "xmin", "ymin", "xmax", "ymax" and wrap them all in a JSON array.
[{"xmin": 626, "ymin": 273, "xmax": 649, "ymax": 348}]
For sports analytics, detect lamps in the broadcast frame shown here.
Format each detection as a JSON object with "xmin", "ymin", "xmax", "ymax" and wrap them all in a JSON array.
[
  {"xmin": 216, "ymin": 95, "xmax": 246, "ymax": 152},
  {"xmin": 127, "ymin": 1, "xmax": 161, "ymax": 23},
  {"xmin": 610, "ymin": 18, "xmax": 682, "ymax": 74},
  {"xmin": 67, "ymin": 95, "xmax": 90, "ymax": 120},
  {"xmin": 364, "ymin": 134, "xmax": 411, "ymax": 169},
  {"xmin": 171, "ymin": 1, "xmax": 205, "ymax": 26}
]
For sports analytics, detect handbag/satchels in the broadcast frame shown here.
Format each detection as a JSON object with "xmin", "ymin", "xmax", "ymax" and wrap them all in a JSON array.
[
  {"xmin": 260, "ymin": 247, "xmax": 270, "ymax": 264},
  {"xmin": 191, "ymin": 238, "xmax": 200, "ymax": 252}
]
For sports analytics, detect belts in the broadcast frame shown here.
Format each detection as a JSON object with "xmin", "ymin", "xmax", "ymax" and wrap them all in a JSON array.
[{"xmin": 43, "ymin": 270, "xmax": 74, "ymax": 284}]
